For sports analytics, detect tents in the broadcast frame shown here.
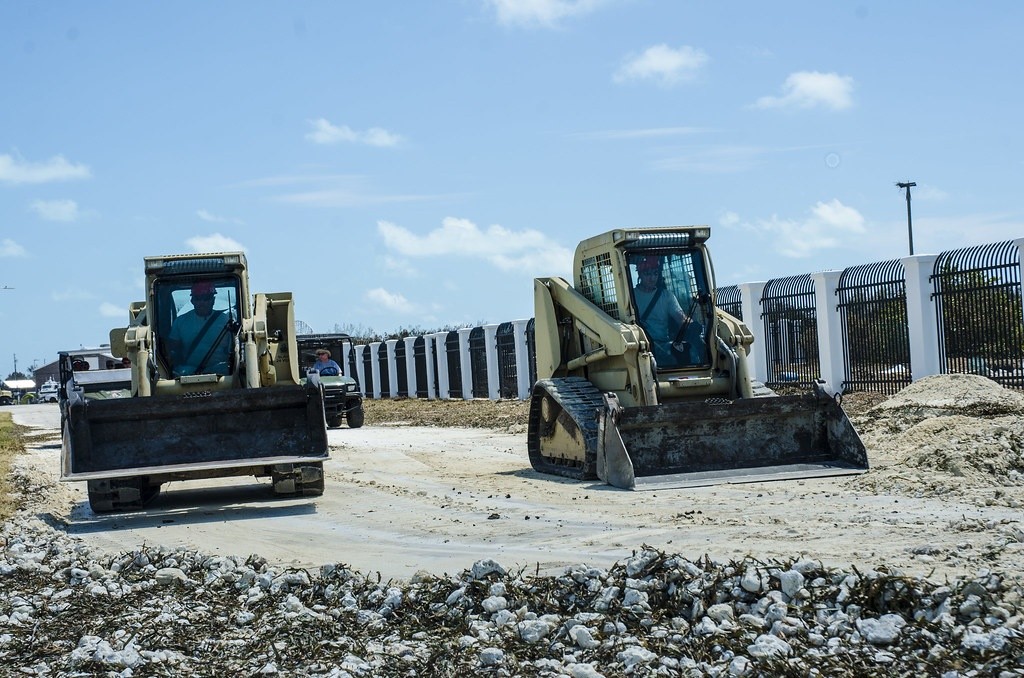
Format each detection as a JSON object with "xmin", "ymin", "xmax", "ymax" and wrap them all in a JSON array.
[{"xmin": 3, "ymin": 380, "xmax": 36, "ymax": 405}]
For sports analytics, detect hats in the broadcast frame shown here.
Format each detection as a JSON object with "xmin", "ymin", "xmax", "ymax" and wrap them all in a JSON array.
[{"xmin": 316, "ymin": 347, "xmax": 332, "ymax": 357}]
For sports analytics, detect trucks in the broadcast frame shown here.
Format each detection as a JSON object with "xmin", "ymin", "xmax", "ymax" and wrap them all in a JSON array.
[{"xmin": 37, "ymin": 381, "xmax": 58, "ymax": 403}]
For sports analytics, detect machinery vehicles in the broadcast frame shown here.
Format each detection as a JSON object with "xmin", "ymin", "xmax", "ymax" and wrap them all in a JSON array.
[
  {"xmin": 525, "ymin": 225, "xmax": 869, "ymax": 493},
  {"xmin": 0, "ymin": 387, "xmax": 12, "ymax": 406},
  {"xmin": 59, "ymin": 249, "xmax": 332, "ymax": 512},
  {"xmin": 56, "ymin": 346, "xmax": 132, "ymax": 440}
]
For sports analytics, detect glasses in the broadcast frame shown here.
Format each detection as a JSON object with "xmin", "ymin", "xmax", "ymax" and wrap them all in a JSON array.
[
  {"xmin": 194, "ymin": 296, "xmax": 211, "ymax": 301},
  {"xmin": 123, "ymin": 362, "xmax": 131, "ymax": 364},
  {"xmin": 106, "ymin": 365, "xmax": 114, "ymax": 367},
  {"xmin": 317, "ymin": 352, "xmax": 325, "ymax": 356}
]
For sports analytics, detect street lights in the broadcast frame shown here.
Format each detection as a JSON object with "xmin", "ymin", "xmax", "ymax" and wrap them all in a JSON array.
[{"xmin": 898, "ymin": 182, "xmax": 916, "ymax": 255}]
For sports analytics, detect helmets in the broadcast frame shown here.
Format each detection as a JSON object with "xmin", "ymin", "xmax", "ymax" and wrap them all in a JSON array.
[
  {"xmin": 190, "ymin": 279, "xmax": 218, "ymax": 295},
  {"xmin": 636, "ymin": 255, "xmax": 661, "ymax": 272}
]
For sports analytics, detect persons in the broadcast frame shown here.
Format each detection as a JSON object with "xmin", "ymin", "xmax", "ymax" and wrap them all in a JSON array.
[
  {"xmin": 163, "ymin": 280, "xmax": 235, "ymax": 379},
  {"xmin": 106, "ymin": 360, "xmax": 115, "ymax": 369},
  {"xmin": 122, "ymin": 358, "xmax": 131, "ymax": 368},
  {"xmin": 312, "ymin": 348, "xmax": 342, "ymax": 376},
  {"xmin": 633, "ymin": 255, "xmax": 694, "ymax": 368},
  {"xmin": 74, "ymin": 362, "xmax": 81, "ymax": 371},
  {"xmin": 80, "ymin": 361, "xmax": 89, "ymax": 371}
]
